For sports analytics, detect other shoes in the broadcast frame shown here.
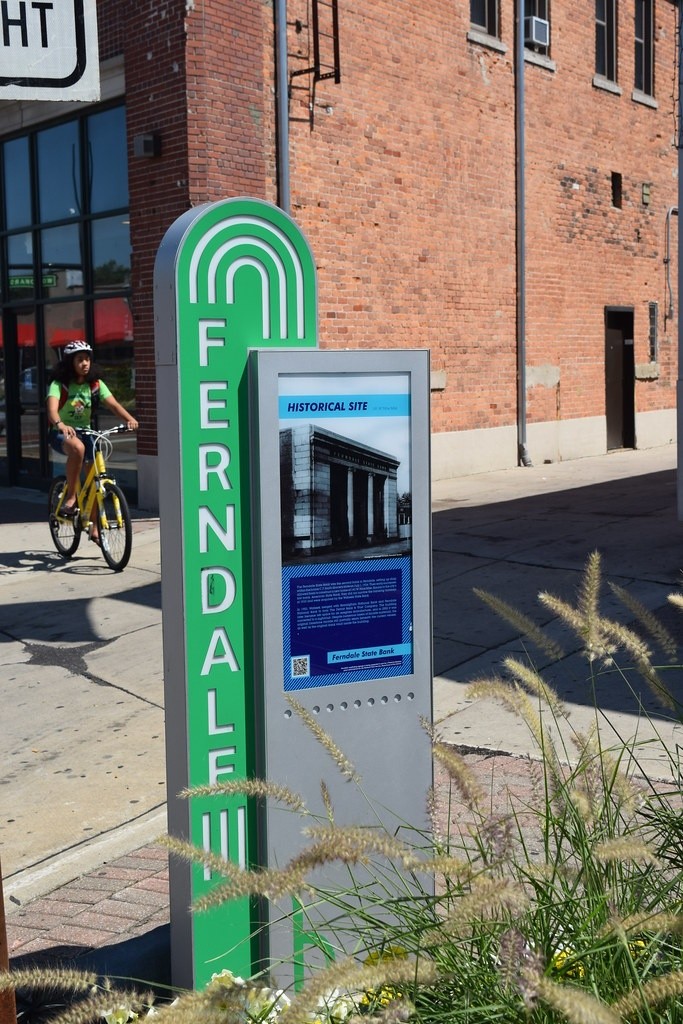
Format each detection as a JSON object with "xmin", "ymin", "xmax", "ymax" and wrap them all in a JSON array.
[
  {"xmin": 64, "ymin": 503, "xmax": 77, "ymax": 515},
  {"xmin": 92, "ymin": 536, "xmax": 99, "ymax": 543}
]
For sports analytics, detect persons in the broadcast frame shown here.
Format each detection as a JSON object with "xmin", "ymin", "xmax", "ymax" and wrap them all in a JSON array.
[{"xmin": 46, "ymin": 340, "xmax": 140, "ymax": 542}]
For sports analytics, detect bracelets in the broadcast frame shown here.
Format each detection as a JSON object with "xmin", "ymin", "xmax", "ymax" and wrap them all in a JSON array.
[{"xmin": 55, "ymin": 420, "xmax": 62, "ymax": 426}]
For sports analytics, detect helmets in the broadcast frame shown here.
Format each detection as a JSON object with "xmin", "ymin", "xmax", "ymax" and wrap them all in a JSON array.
[{"xmin": 64, "ymin": 340, "xmax": 92, "ymax": 354}]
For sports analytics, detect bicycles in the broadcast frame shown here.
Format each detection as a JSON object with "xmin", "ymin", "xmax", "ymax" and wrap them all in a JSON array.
[{"xmin": 45, "ymin": 424, "xmax": 136, "ymax": 572}]
